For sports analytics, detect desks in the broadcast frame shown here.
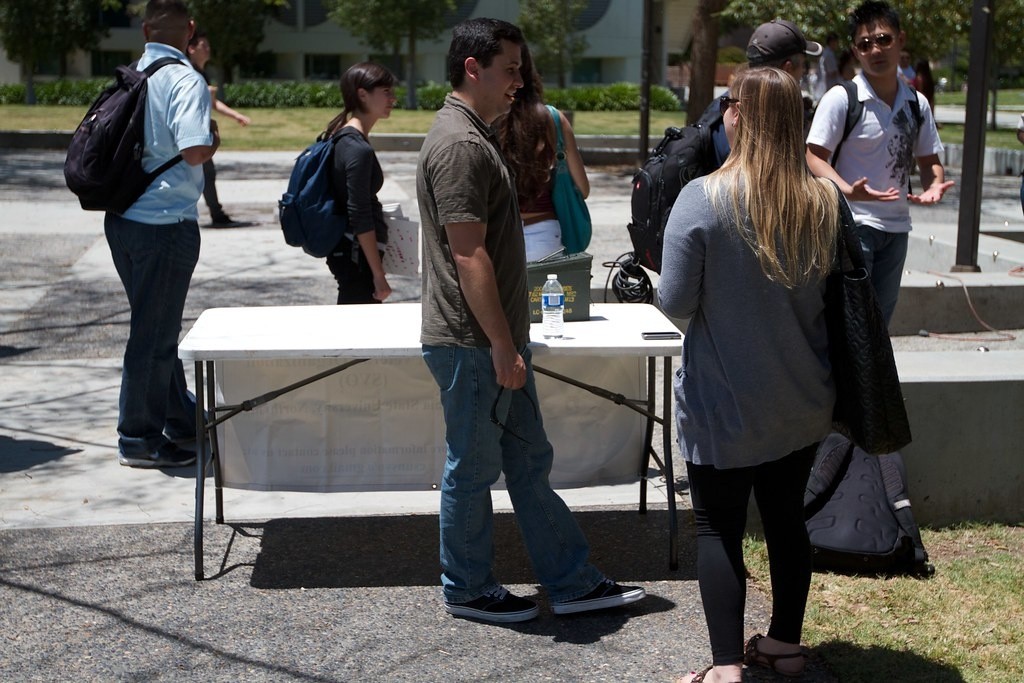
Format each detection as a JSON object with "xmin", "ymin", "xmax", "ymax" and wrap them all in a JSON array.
[{"xmin": 177, "ymin": 303, "xmax": 685, "ymax": 582}]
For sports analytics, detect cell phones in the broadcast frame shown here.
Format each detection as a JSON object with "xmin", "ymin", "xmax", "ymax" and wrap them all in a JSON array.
[{"xmin": 641, "ymin": 332, "xmax": 682, "ymax": 340}]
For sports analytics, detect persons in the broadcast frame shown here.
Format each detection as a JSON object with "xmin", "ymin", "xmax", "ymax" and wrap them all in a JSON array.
[
  {"xmin": 325, "ymin": 61, "xmax": 398, "ymax": 305},
  {"xmin": 1016, "ymin": 115, "xmax": 1024, "ymax": 216},
  {"xmin": 698, "ymin": 19, "xmax": 823, "ymax": 169},
  {"xmin": 488, "ymin": 33, "xmax": 593, "ymax": 263},
  {"xmin": 185, "ymin": 31, "xmax": 250, "ymax": 228},
  {"xmin": 798, "ymin": 1, "xmax": 956, "ymax": 332},
  {"xmin": 418, "ymin": 17, "xmax": 649, "ymax": 623},
  {"xmin": 99, "ymin": 0, "xmax": 220, "ymax": 467},
  {"xmin": 656, "ymin": 67, "xmax": 849, "ymax": 683}
]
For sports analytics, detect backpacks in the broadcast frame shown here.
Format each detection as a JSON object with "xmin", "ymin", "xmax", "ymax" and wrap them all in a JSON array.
[
  {"xmin": 278, "ymin": 127, "xmax": 365, "ymax": 258},
  {"xmin": 63, "ymin": 57, "xmax": 183, "ymax": 215},
  {"xmin": 800, "ymin": 434, "xmax": 936, "ymax": 578},
  {"xmin": 626, "ymin": 101, "xmax": 723, "ymax": 275}
]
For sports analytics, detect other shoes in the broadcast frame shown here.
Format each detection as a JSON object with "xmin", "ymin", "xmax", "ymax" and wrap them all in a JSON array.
[{"xmin": 212, "ymin": 216, "xmax": 239, "ymax": 227}]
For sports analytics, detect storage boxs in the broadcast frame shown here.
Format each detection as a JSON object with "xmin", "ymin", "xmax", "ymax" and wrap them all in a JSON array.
[{"xmin": 526, "ymin": 252, "xmax": 594, "ymax": 323}]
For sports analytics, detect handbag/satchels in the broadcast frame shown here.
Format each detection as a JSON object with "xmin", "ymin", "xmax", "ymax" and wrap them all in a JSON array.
[
  {"xmin": 823, "ymin": 177, "xmax": 912, "ymax": 452},
  {"xmin": 545, "ymin": 104, "xmax": 592, "ymax": 255}
]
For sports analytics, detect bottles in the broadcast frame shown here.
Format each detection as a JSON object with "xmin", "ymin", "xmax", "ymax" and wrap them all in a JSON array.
[{"xmin": 542, "ymin": 274, "xmax": 564, "ymax": 339}]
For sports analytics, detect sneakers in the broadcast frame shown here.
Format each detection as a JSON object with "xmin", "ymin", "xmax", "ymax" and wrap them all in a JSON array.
[
  {"xmin": 444, "ymin": 585, "xmax": 538, "ymax": 622},
  {"xmin": 550, "ymin": 578, "xmax": 647, "ymax": 614},
  {"xmin": 168, "ymin": 411, "xmax": 209, "ymax": 443},
  {"xmin": 118, "ymin": 437, "xmax": 197, "ymax": 467}
]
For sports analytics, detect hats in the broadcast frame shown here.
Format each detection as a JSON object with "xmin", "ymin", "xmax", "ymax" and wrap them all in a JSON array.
[{"xmin": 746, "ymin": 19, "xmax": 823, "ymax": 63}]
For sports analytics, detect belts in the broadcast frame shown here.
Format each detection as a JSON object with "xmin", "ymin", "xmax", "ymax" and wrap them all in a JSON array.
[{"xmin": 521, "ymin": 214, "xmax": 555, "ymax": 226}]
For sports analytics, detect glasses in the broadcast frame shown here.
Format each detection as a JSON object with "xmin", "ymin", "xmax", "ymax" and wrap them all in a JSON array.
[
  {"xmin": 853, "ymin": 33, "xmax": 897, "ymax": 55},
  {"xmin": 489, "ymin": 386, "xmax": 538, "ymax": 445},
  {"xmin": 719, "ymin": 96, "xmax": 740, "ymax": 116}
]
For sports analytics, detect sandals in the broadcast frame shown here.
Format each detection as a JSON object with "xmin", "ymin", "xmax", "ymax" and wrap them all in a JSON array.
[
  {"xmin": 676, "ymin": 666, "xmax": 748, "ymax": 683},
  {"xmin": 743, "ymin": 634, "xmax": 805, "ymax": 677}
]
